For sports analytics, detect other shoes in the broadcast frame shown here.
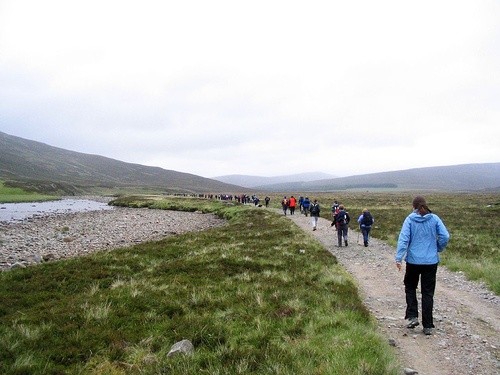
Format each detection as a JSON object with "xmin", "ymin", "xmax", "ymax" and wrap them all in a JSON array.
[
  {"xmin": 345, "ymin": 240, "xmax": 348, "ymax": 246},
  {"xmin": 313, "ymin": 227, "xmax": 317, "ymax": 231},
  {"xmin": 364, "ymin": 241, "xmax": 368, "ymax": 247}
]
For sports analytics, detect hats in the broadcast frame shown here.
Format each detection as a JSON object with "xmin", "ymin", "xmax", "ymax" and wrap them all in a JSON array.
[{"xmin": 339, "ymin": 205, "xmax": 344, "ymax": 209}]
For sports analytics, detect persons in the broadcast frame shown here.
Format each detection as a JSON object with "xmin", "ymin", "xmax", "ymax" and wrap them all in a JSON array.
[
  {"xmin": 357, "ymin": 208, "xmax": 374, "ymax": 247},
  {"xmin": 394, "ymin": 197, "xmax": 450, "ymax": 334},
  {"xmin": 309, "ymin": 200, "xmax": 320, "ymax": 231},
  {"xmin": 328, "ymin": 204, "xmax": 351, "ymax": 247},
  {"xmin": 174, "ymin": 192, "xmax": 310, "ymax": 217},
  {"xmin": 331, "ymin": 200, "xmax": 340, "ymax": 231}
]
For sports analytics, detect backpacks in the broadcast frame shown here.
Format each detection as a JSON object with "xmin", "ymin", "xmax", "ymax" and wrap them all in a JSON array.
[
  {"xmin": 312, "ymin": 204, "xmax": 318, "ymax": 215},
  {"xmin": 362, "ymin": 211, "xmax": 373, "ymax": 226},
  {"xmin": 334, "ymin": 204, "xmax": 339, "ymax": 215},
  {"xmin": 338, "ymin": 212, "xmax": 347, "ymax": 225}
]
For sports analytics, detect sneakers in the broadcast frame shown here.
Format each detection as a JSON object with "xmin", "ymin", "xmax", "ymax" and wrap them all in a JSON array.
[
  {"xmin": 406, "ymin": 316, "xmax": 420, "ymax": 328},
  {"xmin": 423, "ymin": 323, "xmax": 435, "ymax": 335}
]
